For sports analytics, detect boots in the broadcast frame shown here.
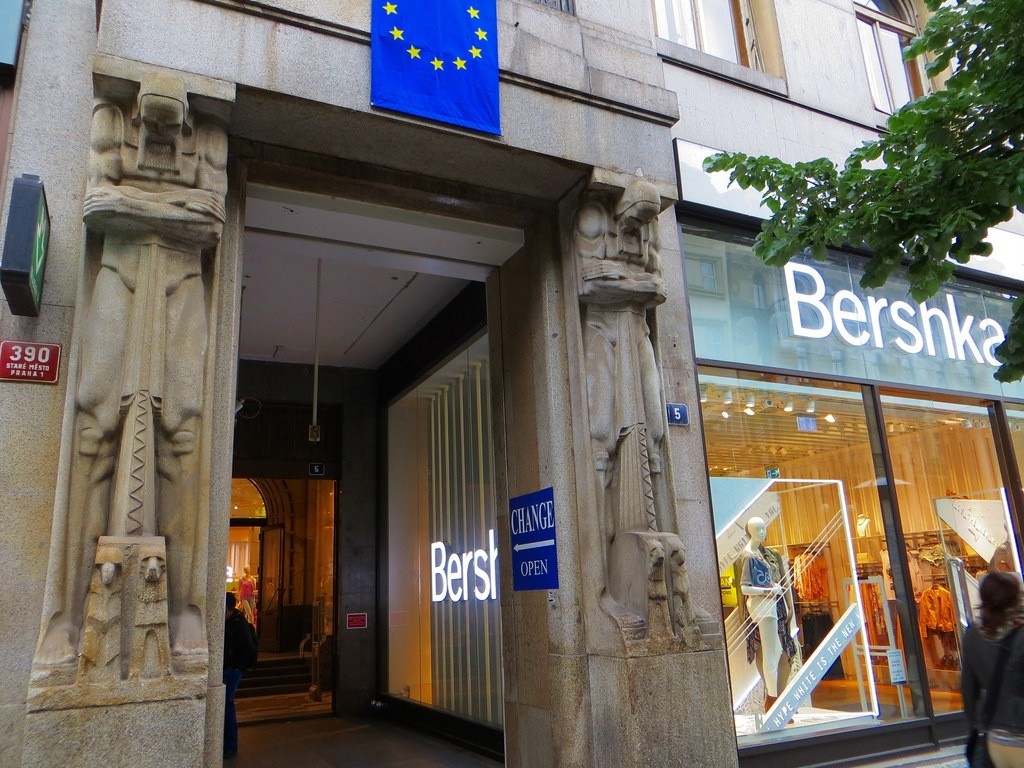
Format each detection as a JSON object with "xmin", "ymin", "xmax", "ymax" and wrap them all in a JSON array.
[{"xmin": 765, "ymin": 695, "xmax": 797, "ymax": 725}]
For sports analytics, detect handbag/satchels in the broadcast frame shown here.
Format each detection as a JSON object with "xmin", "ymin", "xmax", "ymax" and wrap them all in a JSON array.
[{"xmin": 961, "ymin": 716, "xmax": 991, "ymax": 768}]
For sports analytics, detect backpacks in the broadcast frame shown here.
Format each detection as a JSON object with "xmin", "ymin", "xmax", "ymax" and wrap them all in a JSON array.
[{"xmin": 236, "ymin": 618, "xmax": 258, "ymax": 672}]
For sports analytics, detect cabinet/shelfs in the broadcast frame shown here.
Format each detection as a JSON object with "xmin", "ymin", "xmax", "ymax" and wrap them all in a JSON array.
[{"xmin": 783, "ymin": 524, "xmax": 970, "ymax": 694}]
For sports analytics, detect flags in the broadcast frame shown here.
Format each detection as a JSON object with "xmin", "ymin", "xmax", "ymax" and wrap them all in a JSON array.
[{"xmin": 366, "ymin": 1, "xmax": 507, "ymax": 139}]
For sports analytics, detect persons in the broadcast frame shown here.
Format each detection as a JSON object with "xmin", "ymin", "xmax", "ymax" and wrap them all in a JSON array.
[
  {"xmin": 574, "ymin": 174, "xmax": 704, "ymax": 645},
  {"xmin": 224, "ymin": 590, "xmax": 251, "ymax": 762},
  {"xmin": 975, "ymin": 543, "xmax": 1020, "ymax": 582},
  {"xmin": 955, "ymin": 567, "xmax": 1024, "ymax": 768},
  {"xmin": 733, "ymin": 522, "xmax": 801, "ymax": 714},
  {"xmin": 234, "ymin": 563, "xmax": 257, "ymax": 626},
  {"xmin": 738, "ymin": 516, "xmax": 798, "ymax": 725},
  {"xmin": 55, "ymin": 60, "xmax": 216, "ymax": 683}
]
